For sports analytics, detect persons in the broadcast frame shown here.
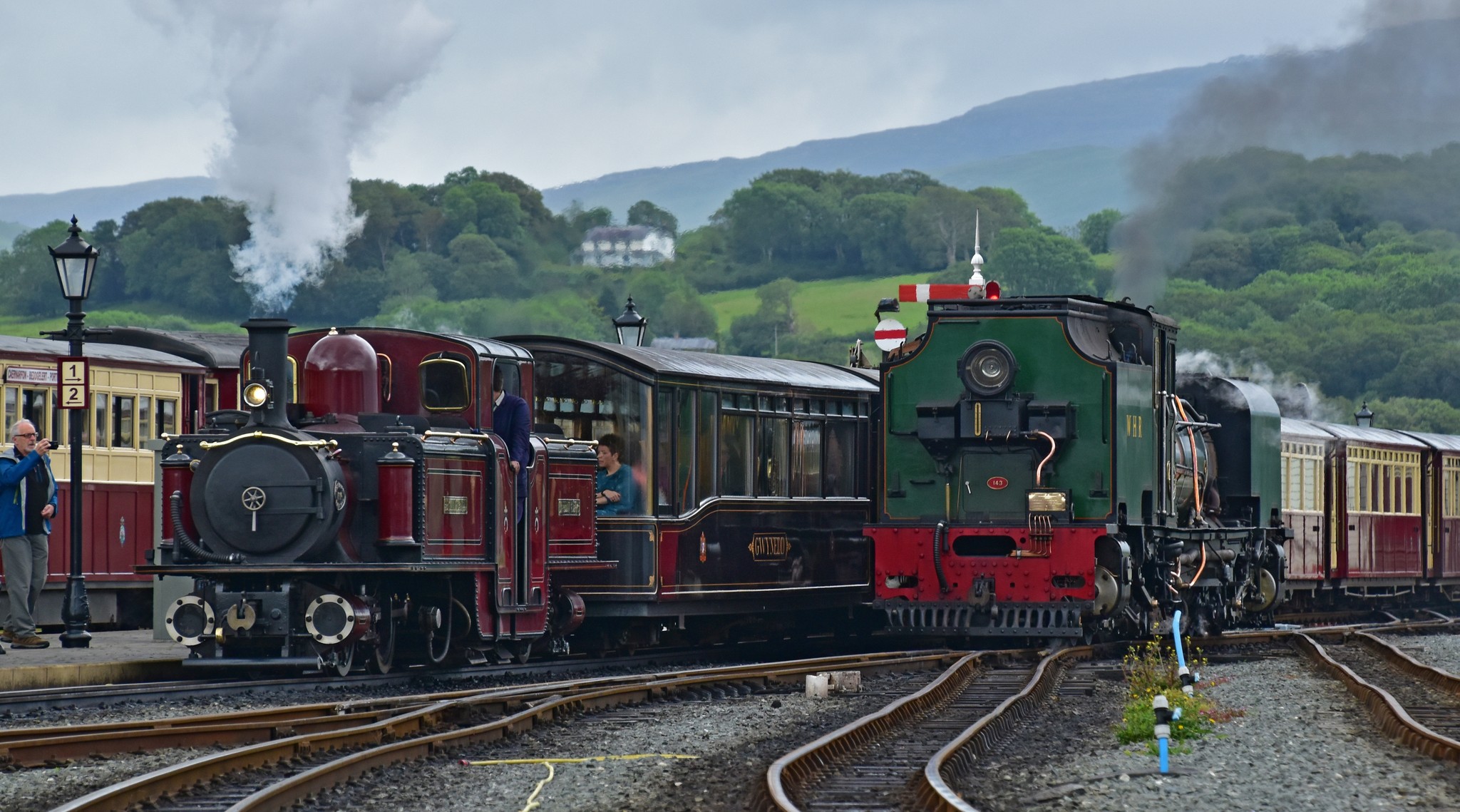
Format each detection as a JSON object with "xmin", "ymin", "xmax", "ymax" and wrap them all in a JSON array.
[
  {"xmin": 493, "ymin": 366, "xmax": 531, "ymax": 522},
  {"xmin": 0, "ymin": 420, "xmax": 59, "ymax": 650},
  {"xmin": 595, "ymin": 433, "xmax": 634, "ymax": 517}
]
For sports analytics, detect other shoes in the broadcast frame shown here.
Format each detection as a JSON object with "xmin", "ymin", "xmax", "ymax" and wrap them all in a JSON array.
[
  {"xmin": 11, "ymin": 635, "xmax": 50, "ymax": 649},
  {"xmin": 0, "ymin": 631, "xmax": 14, "ymax": 643}
]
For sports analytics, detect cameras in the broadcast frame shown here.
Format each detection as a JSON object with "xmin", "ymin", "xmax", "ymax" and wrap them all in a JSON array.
[{"xmin": 47, "ymin": 441, "xmax": 59, "ymax": 450}]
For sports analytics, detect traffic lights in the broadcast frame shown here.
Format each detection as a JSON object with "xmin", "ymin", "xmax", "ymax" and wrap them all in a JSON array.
[{"xmin": 985, "ymin": 279, "xmax": 1001, "ymax": 299}]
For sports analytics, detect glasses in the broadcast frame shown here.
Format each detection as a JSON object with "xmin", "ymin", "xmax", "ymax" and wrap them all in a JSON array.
[{"xmin": 14, "ymin": 432, "xmax": 39, "ymax": 439}]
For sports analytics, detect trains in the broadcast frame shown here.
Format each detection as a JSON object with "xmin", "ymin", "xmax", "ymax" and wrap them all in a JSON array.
[
  {"xmin": 862, "ymin": 293, "xmax": 1460, "ymax": 647},
  {"xmin": 0, "ymin": 324, "xmax": 250, "ymax": 634},
  {"xmin": 131, "ymin": 316, "xmax": 882, "ymax": 678}
]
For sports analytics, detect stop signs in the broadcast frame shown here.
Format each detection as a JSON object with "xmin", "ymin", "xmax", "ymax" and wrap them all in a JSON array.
[{"xmin": 874, "ymin": 319, "xmax": 906, "ymax": 352}]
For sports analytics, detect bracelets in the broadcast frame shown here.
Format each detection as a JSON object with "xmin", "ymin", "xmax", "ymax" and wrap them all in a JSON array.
[
  {"xmin": 604, "ymin": 496, "xmax": 611, "ymax": 504},
  {"xmin": 601, "ymin": 491, "xmax": 605, "ymax": 496}
]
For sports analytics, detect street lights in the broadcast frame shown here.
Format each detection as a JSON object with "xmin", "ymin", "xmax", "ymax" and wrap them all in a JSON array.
[{"xmin": 38, "ymin": 213, "xmax": 113, "ymax": 649}]
